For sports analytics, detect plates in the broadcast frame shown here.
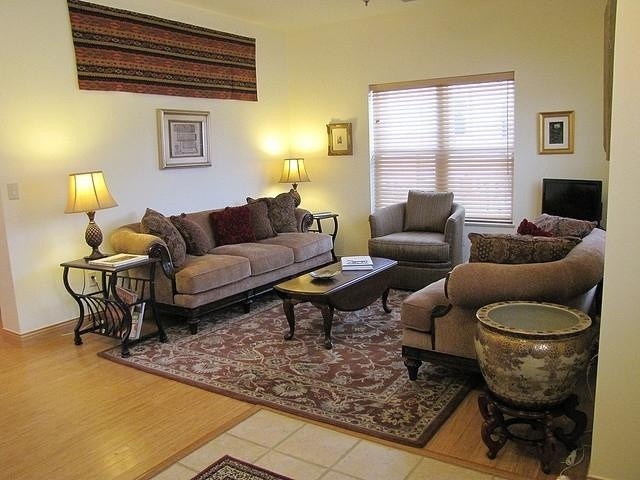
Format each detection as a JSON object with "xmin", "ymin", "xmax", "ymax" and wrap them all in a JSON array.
[{"xmin": 308, "ymin": 268, "xmax": 343, "ymax": 280}]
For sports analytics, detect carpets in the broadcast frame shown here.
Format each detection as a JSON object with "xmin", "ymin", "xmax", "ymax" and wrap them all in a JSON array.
[
  {"xmin": 189, "ymin": 454, "xmax": 293, "ymax": 479},
  {"xmin": 96, "ymin": 289, "xmax": 477, "ymax": 448}
]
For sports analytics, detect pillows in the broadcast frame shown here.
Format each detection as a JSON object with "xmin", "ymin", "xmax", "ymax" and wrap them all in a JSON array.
[
  {"xmin": 404, "ymin": 189, "xmax": 454, "ymax": 232},
  {"xmin": 517, "ymin": 218, "xmax": 553, "ymax": 237},
  {"xmin": 468, "ymin": 233, "xmax": 582, "ymax": 264},
  {"xmin": 140, "ymin": 208, "xmax": 186, "ymax": 274},
  {"xmin": 246, "ymin": 189, "xmax": 297, "ymax": 233},
  {"xmin": 226, "ymin": 198, "xmax": 278, "ymax": 241},
  {"xmin": 528, "ymin": 213, "xmax": 559, "ymax": 235},
  {"xmin": 170, "ymin": 213, "xmax": 210, "ymax": 256},
  {"xmin": 209, "ymin": 206, "xmax": 257, "ymax": 247},
  {"xmin": 559, "ymin": 213, "xmax": 598, "ymax": 238}
]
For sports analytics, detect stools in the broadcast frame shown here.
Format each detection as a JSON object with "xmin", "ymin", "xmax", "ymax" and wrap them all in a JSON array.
[{"xmin": 480, "ymin": 388, "xmax": 587, "ymax": 473}]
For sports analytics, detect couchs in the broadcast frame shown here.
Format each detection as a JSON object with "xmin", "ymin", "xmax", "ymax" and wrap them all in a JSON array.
[
  {"xmin": 110, "ymin": 192, "xmax": 334, "ymax": 335},
  {"xmin": 400, "ymin": 214, "xmax": 606, "ymax": 381},
  {"xmin": 367, "ymin": 189, "xmax": 465, "ymax": 292}
]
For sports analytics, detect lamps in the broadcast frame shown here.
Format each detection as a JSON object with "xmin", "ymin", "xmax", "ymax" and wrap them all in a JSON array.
[
  {"xmin": 65, "ymin": 171, "xmax": 118, "ymax": 260},
  {"xmin": 278, "ymin": 158, "xmax": 311, "ymax": 208}
]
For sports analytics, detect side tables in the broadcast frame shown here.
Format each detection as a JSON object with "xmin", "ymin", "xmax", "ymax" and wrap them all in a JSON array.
[
  {"xmin": 60, "ymin": 254, "xmax": 169, "ymax": 359},
  {"xmin": 308, "ymin": 212, "xmax": 339, "ymax": 261}
]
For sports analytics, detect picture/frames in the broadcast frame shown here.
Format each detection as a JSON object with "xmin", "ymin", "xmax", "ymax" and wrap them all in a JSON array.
[
  {"xmin": 326, "ymin": 123, "xmax": 353, "ymax": 156},
  {"xmin": 156, "ymin": 108, "xmax": 212, "ymax": 170},
  {"xmin": 539, "ymin": 110, "xmax": 574, "ymax": 154}
]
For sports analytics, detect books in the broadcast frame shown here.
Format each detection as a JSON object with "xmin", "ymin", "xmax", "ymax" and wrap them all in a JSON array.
[
  {"xmin": 104, "ymin": 285, "xmax": 145, "ymax": 340},
  {"xmin": 342, "ymin": 255, "xmax": 374, "ymax": 271}
]
